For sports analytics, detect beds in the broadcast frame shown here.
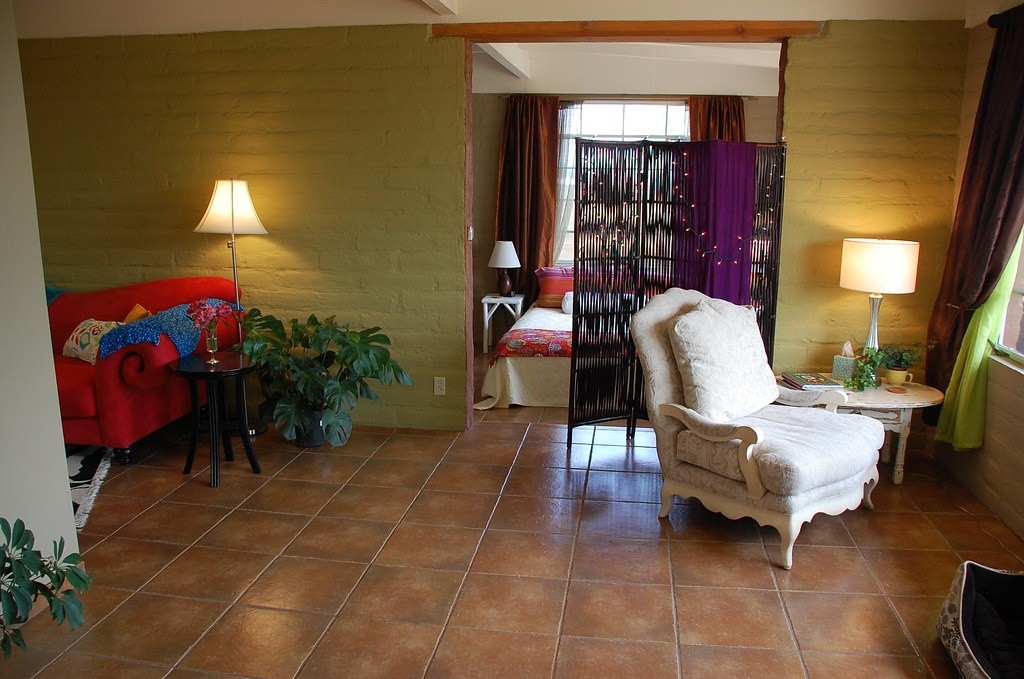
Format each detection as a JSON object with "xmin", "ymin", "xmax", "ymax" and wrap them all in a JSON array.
[{"xmin": 498, "ymin": 266, "xmax": 687, "ymax": 405}]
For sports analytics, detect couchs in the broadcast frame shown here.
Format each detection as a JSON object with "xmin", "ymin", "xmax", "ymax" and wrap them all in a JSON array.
[{"xmin": 44, "ymin": 276, "xmax": 247, "ymax": 464}]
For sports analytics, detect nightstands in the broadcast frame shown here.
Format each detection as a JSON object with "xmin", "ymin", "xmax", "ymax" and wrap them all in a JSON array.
[{"xmin": 481, "ymin": 293, "xmax": 525, "ymax": 354}]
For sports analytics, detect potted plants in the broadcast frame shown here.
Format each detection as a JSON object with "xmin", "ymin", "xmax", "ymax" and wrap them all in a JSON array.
[
  {"xmin": 845, "ymin": 337, "xmax": 940, "ymax": 393},
  {"xmin": 234, "ymin": 308, "xmax": 415, "ymax": 448}
]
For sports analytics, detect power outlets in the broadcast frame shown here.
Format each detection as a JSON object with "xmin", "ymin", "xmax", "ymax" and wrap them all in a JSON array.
[{"xmin": 434, "ymin": 377, "xmax": 445, "ymax": 395}]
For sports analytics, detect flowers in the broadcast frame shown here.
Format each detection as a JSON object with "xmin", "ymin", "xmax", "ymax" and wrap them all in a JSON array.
[{"xmin": 185, "ymin": 296, "xmax": 232, "ymax": 330}]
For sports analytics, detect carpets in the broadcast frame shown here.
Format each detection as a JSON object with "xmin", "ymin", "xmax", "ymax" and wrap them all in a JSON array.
[{"xmin": 64, "ymin": 443, "xmax": 111, "ymax": 533}]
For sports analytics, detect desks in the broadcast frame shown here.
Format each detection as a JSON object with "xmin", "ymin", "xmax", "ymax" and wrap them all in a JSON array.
[
  {"xmin": 166, "ymin": 352, "xmax": 263, "ymax": 487},
  {"xmin": 775, "ymin": 373, "xmax": 944, "ymax": 484}
]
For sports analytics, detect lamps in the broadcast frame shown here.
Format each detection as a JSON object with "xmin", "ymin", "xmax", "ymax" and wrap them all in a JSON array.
[
  {"xmin": 193, "ymin": 178, "xmax": 272, "ymax": 436},
  {"xmin": 838, "ymin": 238, "xmax": 919, "ymax": 356},
  {"xmin": 487, "ymin": 241, "xmax": 521, "ymax": 297}
]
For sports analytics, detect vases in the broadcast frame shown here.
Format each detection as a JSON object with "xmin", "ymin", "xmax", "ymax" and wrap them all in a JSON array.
[{"xmin": 206, "ymin": 325, "xmax": 220, "ymax": 364}]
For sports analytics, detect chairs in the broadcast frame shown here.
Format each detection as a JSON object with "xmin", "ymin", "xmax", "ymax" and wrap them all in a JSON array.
[{"xmin": 631, "ymin": 287, "xmax": 886, "ymax": 570}]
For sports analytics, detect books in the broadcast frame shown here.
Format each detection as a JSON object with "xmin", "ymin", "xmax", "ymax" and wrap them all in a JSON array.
[{"xmin": 777, "ymin": 372, "xmax": 844, "ymax": 391}]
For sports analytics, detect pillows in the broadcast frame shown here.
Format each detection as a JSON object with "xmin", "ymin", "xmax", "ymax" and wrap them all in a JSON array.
[
  {"xmin": 63, "ymin": 318, "xmax": 127, "ymax": 366},
  {"xmin": 534, "ymin": 268, "xmax": 681, "ymax": 314},
  {"xmin": 666, "ymin": 297, "xmax": 779, "ymax": 423}
]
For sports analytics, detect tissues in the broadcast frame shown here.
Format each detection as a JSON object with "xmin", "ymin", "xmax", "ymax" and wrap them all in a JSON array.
[{"xmin": 832, "ymin": 340, "xmax": 863, "ymax": 382}]
